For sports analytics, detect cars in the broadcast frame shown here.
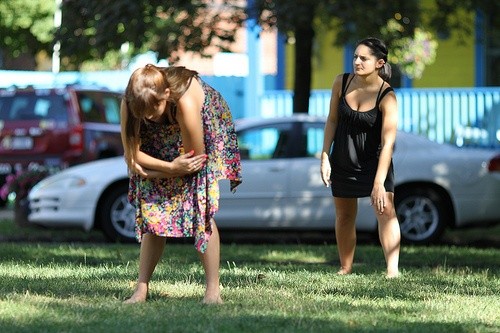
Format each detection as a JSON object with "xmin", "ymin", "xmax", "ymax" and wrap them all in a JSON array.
[{"xmin": 27, "ymin": 113, "xmax": 500, "ymax": 247}]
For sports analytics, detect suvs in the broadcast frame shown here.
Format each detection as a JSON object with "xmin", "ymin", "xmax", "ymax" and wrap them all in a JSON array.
[{"xmin": 0, "ymin": 87, "xmax": 130, "ymax": 210}]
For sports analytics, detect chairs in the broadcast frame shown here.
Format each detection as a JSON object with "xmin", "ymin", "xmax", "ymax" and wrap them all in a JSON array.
[{"xmin": 271, "ymin": 128, "xmax": 309, "ymax": 161}]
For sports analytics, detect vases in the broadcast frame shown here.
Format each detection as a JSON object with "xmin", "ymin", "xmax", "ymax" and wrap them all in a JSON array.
[{"xmin": 11, "ymin": 199, "xmax": 38, "ymax": 227}]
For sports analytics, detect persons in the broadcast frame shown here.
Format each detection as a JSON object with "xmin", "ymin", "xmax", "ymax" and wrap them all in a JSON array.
[
  {"xmin": 120, "ymin": 62, "xmax": 241, "ymax": 305},
  {"xmin": 320, "ymin": 36, "xmax": 401, "ymax": 279}
]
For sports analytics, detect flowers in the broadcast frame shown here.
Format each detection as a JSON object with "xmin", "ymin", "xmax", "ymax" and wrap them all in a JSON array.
[{"xmin": 0, "ymin": 165, "xmax": 59, "ymax": 207}]
